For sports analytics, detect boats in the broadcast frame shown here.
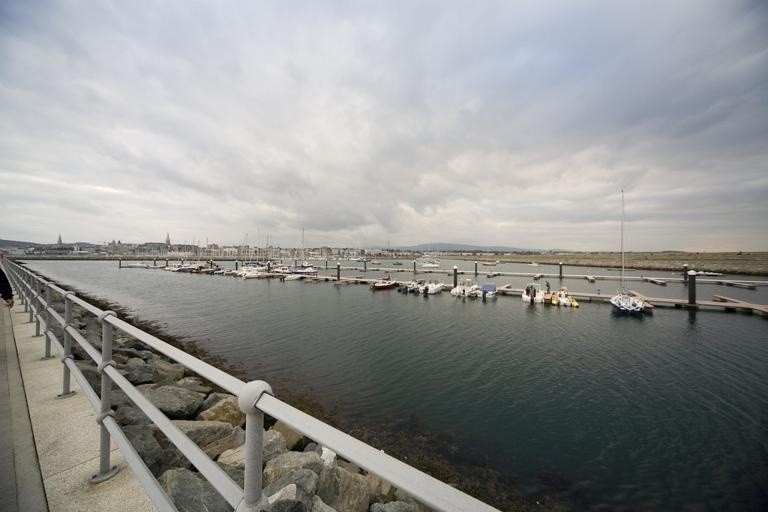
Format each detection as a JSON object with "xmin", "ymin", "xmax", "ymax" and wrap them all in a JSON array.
[
  {"xmin": 522, "ymin": 283, "xmax": 579, "ymax": 307},
  {"xmin": 349, "ymin": 254, "xmax": 543, "ymax": 267},
  {"xmin": 371, "ymin": 280, "xmax": 498, "ymax": 299},
  {"xmin": 610, "ymin": 293, "xmax": 644, "ymax": 314}
]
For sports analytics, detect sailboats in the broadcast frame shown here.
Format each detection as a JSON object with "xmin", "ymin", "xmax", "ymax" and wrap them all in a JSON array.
[{"xmin": 164, "ymin": 228, "xmax": 318, "ymax": 279}]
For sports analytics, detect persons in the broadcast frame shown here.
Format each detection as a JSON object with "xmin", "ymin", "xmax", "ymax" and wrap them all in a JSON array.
[
  {"xmin": 0, "ymin": 268, "xmax": 14, "ymax": 309},
  {"xmin": 545, "ymin": 281, "xmax": 551, "ymax": 293},
  {"xmin": 526, "ymin": 285, "xmax": 536, "ymax": 297}
]
